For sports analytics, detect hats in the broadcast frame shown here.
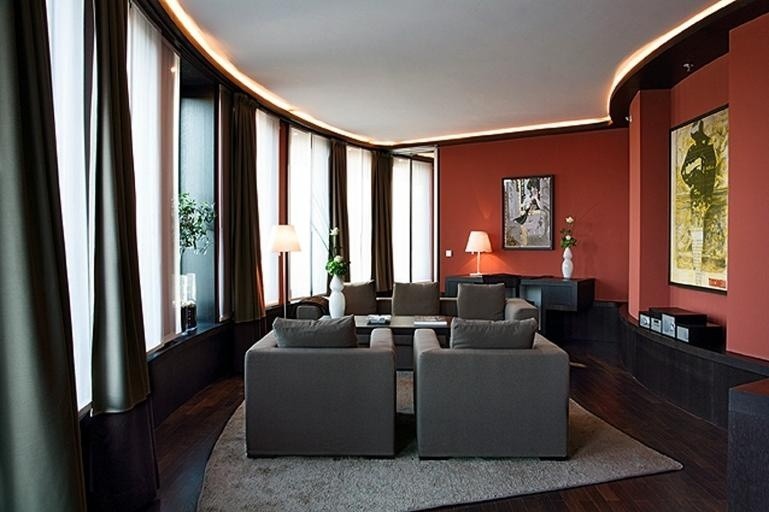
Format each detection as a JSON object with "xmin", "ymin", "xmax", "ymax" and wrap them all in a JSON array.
[{"xmin": 526, "ymin": 178, "xmax": 540, "ymax": 190}]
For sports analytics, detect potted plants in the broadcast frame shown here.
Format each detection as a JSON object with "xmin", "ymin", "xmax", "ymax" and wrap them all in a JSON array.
[{"xmin": 178, "ymin": 191, "xmax": 216, "ymax": 335}]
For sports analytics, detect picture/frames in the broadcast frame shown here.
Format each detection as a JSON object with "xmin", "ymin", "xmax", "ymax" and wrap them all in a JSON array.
[
  {"xmin": 502, "ymin": 174, "xmax": 555, "ymax": 249},
  {"xmin": 668, "ymin": 104, "xmax": 729, "ymax": 295}
]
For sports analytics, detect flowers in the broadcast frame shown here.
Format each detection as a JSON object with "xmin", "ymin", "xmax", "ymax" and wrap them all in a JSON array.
[
  {"xmin": 559, "ymin": 216, "xmax": 578, "ymax": 249},
  {"xmin": 324, "ymin": 227, "xmax": 351, "ymax": 275}
]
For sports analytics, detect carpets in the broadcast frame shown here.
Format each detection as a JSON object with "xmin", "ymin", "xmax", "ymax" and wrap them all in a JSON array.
[{"xmin": 197, "ymin": 370, "xmax": 683, "ymax": 512}]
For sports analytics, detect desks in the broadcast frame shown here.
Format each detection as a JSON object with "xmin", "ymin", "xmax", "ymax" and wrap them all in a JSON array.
[
  {"xmin": 318, "ymin": 314, "xmax": 455, "ymax": 336},
  {"xmin": 445, "ymin": 275, "xmax": 596, "ymax": 339}
]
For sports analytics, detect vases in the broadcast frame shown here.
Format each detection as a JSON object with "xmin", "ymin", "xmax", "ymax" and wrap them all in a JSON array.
[
  {"xmin": 561, "ymin": 247, "xmax": 573, "ymax": 278},
  {"xmin": 328, "ymin": 271, "xmax": 347, "ymax": 319}
]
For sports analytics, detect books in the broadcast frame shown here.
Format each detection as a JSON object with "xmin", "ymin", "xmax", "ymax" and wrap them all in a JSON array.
[{"xmin": 416, "ymin": 315, "xmax": 449, "ymax": 325}]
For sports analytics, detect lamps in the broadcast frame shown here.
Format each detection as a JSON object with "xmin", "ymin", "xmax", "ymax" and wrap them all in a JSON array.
[
  {"xmin": 271, "ymin": 224, "xmax": 302, "ymax": 319},
  {"xmin": 465, "ymin": 230, "xmax": 491, "ymax": 276}
]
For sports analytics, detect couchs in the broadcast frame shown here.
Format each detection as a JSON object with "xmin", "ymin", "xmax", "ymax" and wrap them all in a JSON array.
[
  {"xmin": 296, "ymin": 293, "xmax": 540, "ymax": 370},
  {"xmin": 411, "ymin": 329, "xmax": 570, "ymax": 459},
  {"xmin": 244, "ymin": 327, "xmax": 398, "ymax": 459}
]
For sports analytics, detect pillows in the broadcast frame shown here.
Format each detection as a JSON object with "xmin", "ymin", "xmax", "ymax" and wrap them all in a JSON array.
[
  {"xmin": 449, "ymin": 317, "xmax": 538, "ymax": 349},
  {"xmin": 457, "ymin": 284, "xmax": 506, "ymax": 321},
  {"xmin": 273, "ymin": 314, "xmax": 358, "ymax": 350},
  {"xmin": 393, "ymin": 281, "xmax": 440, "ymax": 317},
  {"xmin": 341, "ymin": 280, "xmax": 377, "ymax": 318}
]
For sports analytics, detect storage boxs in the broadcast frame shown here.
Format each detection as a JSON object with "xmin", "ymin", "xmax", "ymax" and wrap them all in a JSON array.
[
  {"xmin": 676, "ymin": 323, "xmax": 722, "ymax": 349},
  {"xmin": 638, "ymin": 311, "xmax": 650, "ymax": 329},
  {"xmin": 649, "ymin": 306, "xmax": 688, "ymax": 334},
  {"xmin": 662, "ymin": 311, "xmax": 709, "ymax": 339}
]
